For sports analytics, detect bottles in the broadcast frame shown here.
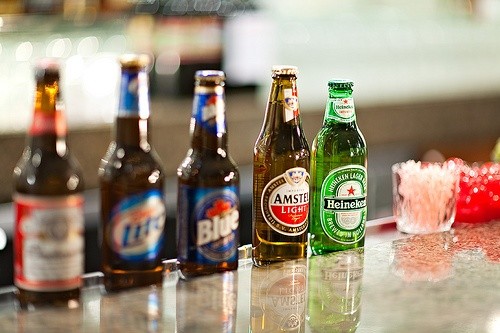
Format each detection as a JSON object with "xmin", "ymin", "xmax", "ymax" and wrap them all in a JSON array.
[
  {"xmin": 11, "ymin": 64, "xmax": 85, "ymax": 310},
  {"xmin": 250, "ymin": 262, "xmax": 307, "ymax": 333},
  {"xmin": 98, "ymin": 53, "xmax": 167, "ymax": 292},
  {"xmin": 307, "ymin": 248, "xmax": 364, "ymax": 333},
  {"xmin": 100, "ymin": 283, "xmax": 167, "ymax": 332},
  {"xmin": 176, "ymin": 270, "xmax": 237, "ymax": 333},
  {"xmin": 308, "ymin": 80, "xmax": 369, "ymax": 255},
  {"xmin": 176, "ymin": 70, "xmax": 241, "ymax": 277},
  {"xmin": 251, "ymin": 65, "xmax": 312, "ymax": 267},
  {"xmin": 16, "ymin": 304, "xmax": 85, "ymax": 332}
]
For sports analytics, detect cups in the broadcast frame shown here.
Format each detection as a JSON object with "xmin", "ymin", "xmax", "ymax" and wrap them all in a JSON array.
[
  {"xmin": 445, "ymin": 157, "xmax": 500, "ymax": 230},
  {"xmin": 393, "ymin": 160, "xmax": 459, "ymax": 235}
]
